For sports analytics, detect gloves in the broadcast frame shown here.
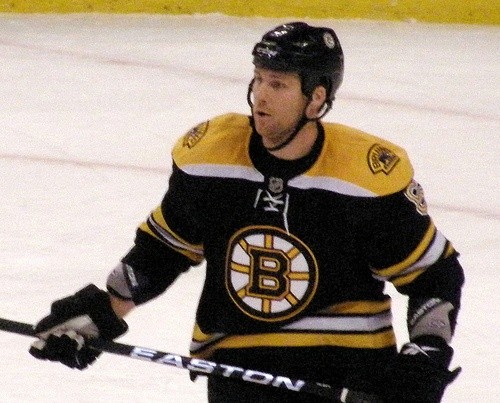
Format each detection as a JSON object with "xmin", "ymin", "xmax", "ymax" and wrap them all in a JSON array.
[
  {"xmin": 385, "ymin": 335, "xmax": 454, "ymax": 403},
  {"xmin": 29, "ymin": 284, "xmax": 128, "ymax": 370}
]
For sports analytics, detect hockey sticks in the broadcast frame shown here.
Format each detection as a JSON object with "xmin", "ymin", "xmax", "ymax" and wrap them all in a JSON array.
[{"xmin": 0, "ymin": 318, "xmax": 379, "ymax": 403}]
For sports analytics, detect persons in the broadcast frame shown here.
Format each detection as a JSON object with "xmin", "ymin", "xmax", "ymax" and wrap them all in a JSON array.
[{"xmin": 30, "ymin": 21, "xmax": 465, "ymax": 402}]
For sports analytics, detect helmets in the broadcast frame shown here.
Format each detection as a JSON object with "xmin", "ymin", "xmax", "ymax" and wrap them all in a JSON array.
[{"xmin": 253, "ymin": 22, "xmax": 344, "ymax": 105}]
